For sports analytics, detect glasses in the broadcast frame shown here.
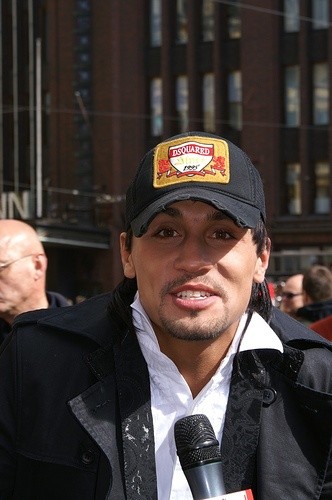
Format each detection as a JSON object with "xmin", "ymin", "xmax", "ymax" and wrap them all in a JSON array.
[{"xmin": 283, "ymin": 291, "xmax": 304, "ymax": 299}]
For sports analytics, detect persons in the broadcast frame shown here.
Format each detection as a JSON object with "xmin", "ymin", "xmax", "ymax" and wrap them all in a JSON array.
[
  {"xmin": 279, "ymin": 266, "xmax": 332, "ymax": 342},
  {"xmin": 0, "ymin": 131, "xmax": 332, "ymax": 500},
  {"xmin": 0, "ymin": 219, "xmax": 68, "ymax": 328}
]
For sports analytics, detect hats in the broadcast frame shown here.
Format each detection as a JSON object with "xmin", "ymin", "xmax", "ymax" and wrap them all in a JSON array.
[{"xmin": 126, "ymin": 131, "xmax": 266, "ymax": 238}]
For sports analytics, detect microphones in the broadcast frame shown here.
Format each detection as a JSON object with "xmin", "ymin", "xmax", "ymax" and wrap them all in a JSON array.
[{"xmin": 174, "ymin": 413, "xmax": 254, "ymax": 500}]
